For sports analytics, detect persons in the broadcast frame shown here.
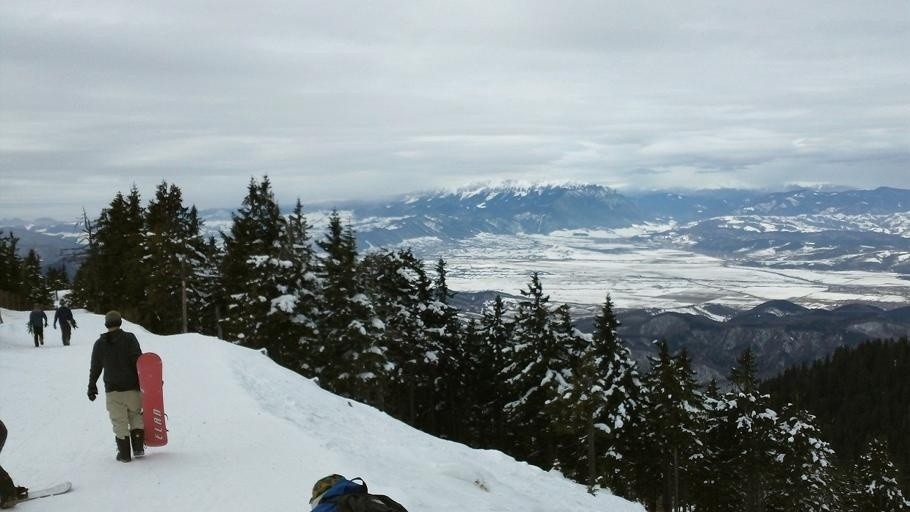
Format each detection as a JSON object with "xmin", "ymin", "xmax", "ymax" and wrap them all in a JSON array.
[
  {"xmin": 54, "ymin": 298, "xmax": 75, "ymax": 347},
  {"xmin": 29, "ymin": 303, "xmax": 48, "ymax": 347},
  {"xmin": 309, "ymin": 473, "xmax": 362, "ymax": 512},
  {"xmin": 85, "ymin": 310, "xmax": 164, "ymax": 461},
  {"xmin": 0, "ymin": 420, "xmax": 29, "ymax": 508}
]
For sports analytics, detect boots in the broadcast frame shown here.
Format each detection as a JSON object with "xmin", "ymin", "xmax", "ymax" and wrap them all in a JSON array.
[
  {"xmin": 130, "ymin": 428, "xmax": 146, "ymax": 457},
  {"xmin": 116, "ymin": 435, "xmax": 131, "ymax": 463}
]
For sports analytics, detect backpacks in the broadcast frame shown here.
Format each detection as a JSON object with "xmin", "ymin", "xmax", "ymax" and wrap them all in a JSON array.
[{"xmin": 319, "ymin": 477, "xmax": 408, "ymax": 512}]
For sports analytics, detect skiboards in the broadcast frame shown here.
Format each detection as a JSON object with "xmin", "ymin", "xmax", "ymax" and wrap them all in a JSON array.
[{"xmin": 0, "ymin": 482, "xmax": 72, "ymax": 506}]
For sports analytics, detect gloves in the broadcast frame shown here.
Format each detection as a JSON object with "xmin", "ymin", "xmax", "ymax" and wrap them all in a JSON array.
[{"xmin": 87, "ymin": 383, "xmax": 99, "ymax": 402}]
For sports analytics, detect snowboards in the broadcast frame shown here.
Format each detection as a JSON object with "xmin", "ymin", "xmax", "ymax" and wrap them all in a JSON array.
[{"xmin": 137, "ymin": 352, "xmax": 168, "ymax": 447}]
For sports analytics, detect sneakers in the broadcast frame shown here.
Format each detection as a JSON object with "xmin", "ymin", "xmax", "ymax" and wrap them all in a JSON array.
[{"xmin": 2, "ymin": 483, "xmax": 29, "ymax": 503}]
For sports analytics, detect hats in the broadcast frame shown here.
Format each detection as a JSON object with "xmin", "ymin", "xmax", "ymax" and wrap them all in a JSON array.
[
  {"xmin": 309, "ymin": 473, "xmax": 345, "ymax": 504},
  {"xmin": 105, "ymin": 310, "xmax": 121, "ymax": 321}
]
